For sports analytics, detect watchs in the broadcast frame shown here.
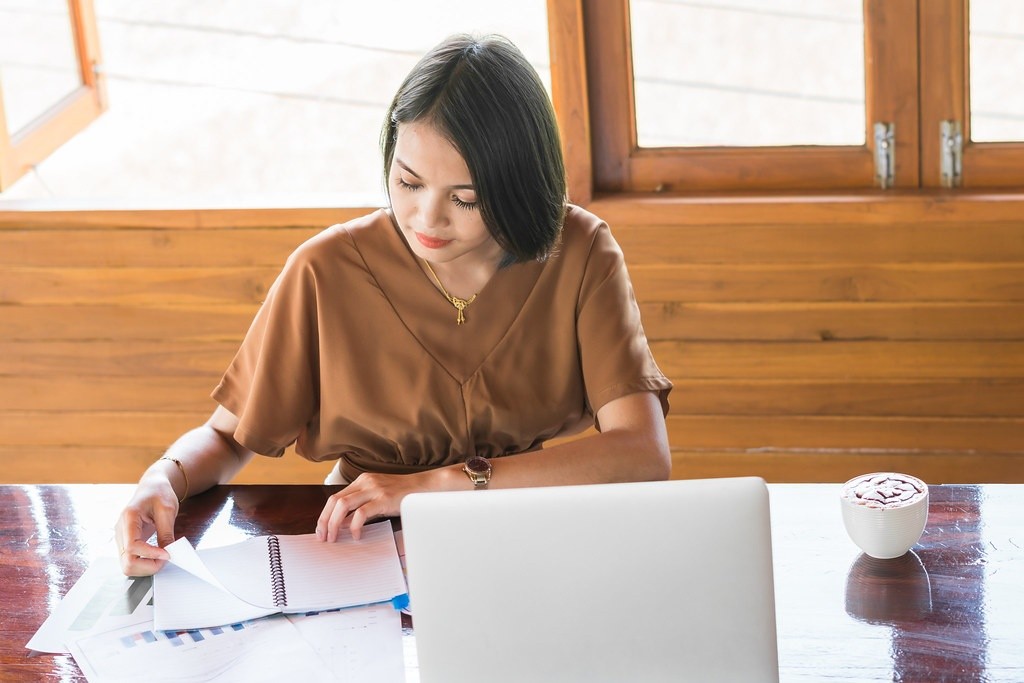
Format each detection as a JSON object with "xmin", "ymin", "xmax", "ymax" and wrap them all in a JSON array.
[{"xmin": 463, "ymin": 456, "xmax": 492, "ymax": 491}]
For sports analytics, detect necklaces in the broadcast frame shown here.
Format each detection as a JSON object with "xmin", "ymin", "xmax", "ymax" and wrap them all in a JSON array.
[{"xmin": 424, "ymin": 260, "xmax": 477, "ymax": 325}]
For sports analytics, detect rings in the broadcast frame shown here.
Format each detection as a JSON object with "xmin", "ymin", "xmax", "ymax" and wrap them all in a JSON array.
[{"xmin": 120, "ymin": 544, "xmax": 124, "ymax": 557}]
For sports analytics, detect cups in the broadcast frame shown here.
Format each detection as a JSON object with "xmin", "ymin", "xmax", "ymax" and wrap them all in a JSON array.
[{"xmin": 840, "ymin": 472, "xmax": 929, "ymax": 559}]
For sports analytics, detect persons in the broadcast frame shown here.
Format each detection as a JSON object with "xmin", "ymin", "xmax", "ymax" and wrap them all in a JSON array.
[{"xmin": 114, "ymin": 33, "xmax": 671, "ymax": 578}]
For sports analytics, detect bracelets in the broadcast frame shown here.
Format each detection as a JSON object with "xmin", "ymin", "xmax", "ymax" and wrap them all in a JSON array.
[{"xmin": 159, "ymin": 456, "xmax": 189, "ymax": 505}]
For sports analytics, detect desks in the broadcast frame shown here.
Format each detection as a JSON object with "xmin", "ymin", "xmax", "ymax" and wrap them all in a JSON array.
[{"xmin": 0, "ymin": 483, "xmax": 1024, "ymax": 683}]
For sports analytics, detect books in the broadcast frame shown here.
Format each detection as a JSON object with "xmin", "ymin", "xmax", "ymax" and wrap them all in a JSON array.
[{"xmin": 152, "ymin": 519, "xmax": 409, "ymax": 633}]
